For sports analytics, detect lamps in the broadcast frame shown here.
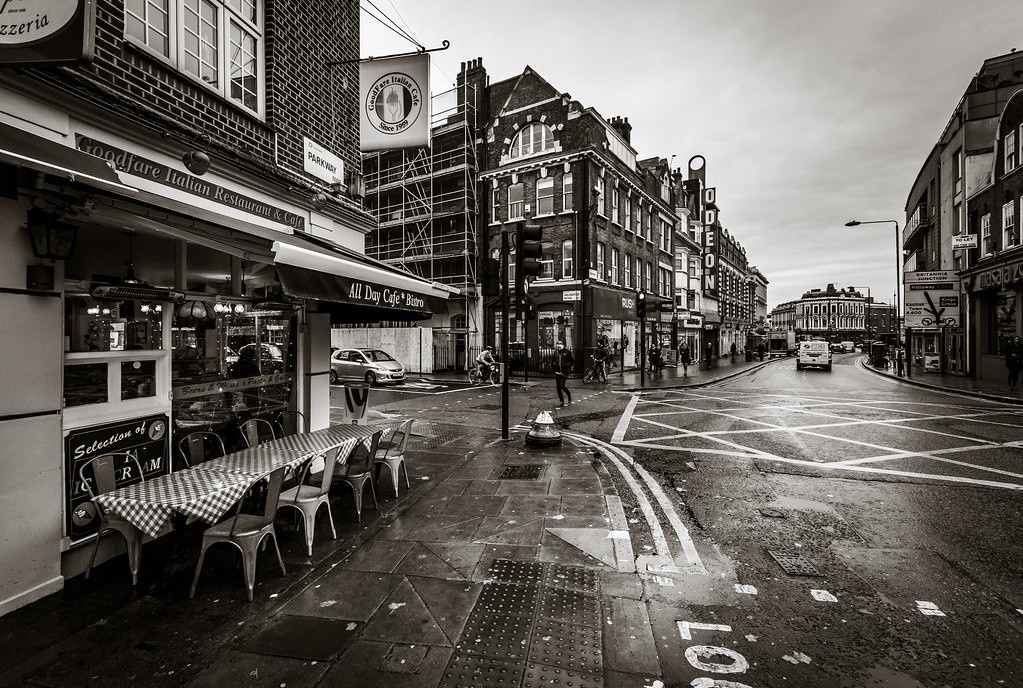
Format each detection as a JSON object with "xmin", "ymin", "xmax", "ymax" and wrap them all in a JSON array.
[
  {"xmin": 181, "ymin": 133, "xmax": 211, "ymax": 175},
  {"xmin": 86, "ymin": 302, "xmax": 244, "ymax": 318},
  {"xmin": 288, "ymin": 179, "xmax": 327, "ymax": 211}
]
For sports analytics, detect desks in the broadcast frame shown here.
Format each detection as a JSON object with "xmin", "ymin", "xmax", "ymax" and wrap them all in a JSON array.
[
  {"xmin": 260, "ymin": 434, "xmax": 358, "ymax": 487},
  {"xmin": 310, "ymin": 423, "xmax": 392, "ymax": 484},
  {"xmin": 189, "ymin": 447, "xmax": 316, "ymax": 531},
  {"xmin": 88, "ymin": 469, "xmax": 255, "ymax": 595}
]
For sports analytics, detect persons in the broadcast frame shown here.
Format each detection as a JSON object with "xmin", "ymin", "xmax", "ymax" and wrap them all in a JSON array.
[
  {"xmin": 1005, "ymin": 336, "xmax": 1023, "ymax": 390},
  {"xmin": 647, "ymin": 342, "xmax": 765, "ymax": 377},
  {"xmin": 550, "ymin": 341, "xmax": 573, "ymax": 407},
  {"xmin": 475, "ymin": 346, "xmax": 495, "ymax": 383},
  {"xmin": 589, "ymin": 333, "xmax": 629, "ymax": 382}
]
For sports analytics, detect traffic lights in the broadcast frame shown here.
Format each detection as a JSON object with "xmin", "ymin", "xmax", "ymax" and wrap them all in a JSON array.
[
  {"xmin": 517, "ymin": 222, "xmax": 544, "ymax": 280},
  {"xmin": 636, "ymin": 292, "xmax": 646, "ymax": 318}
]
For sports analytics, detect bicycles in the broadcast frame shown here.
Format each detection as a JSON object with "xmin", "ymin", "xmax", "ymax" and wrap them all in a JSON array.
[
  {"xmin": 468, "ymin": 362, "xmax": 503, "ymax": 388},
  {"xmin": 582, "ymin": 356, "xmax": 609, "ymax": 385}
]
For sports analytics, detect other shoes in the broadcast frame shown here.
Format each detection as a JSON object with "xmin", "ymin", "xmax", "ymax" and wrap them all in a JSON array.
[
  {"xmin": 683, "ymin": 371, "xmax": 687, "ymax": 376},
  {"xmin": 603, "ymin": 378, "xmax": 608, "ymax": 384},
  {"xmin": 557, "ymin": 401, "xmax": 564, "ymax": 407},
  {"xmin": 568, "ymin": 399, "xmax": 572, "ymax": 404}
]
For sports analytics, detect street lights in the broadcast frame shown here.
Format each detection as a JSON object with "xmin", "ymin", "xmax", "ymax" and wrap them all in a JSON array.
[
  {"xmin": 768, "ymin": 320, "xmax": 772, "ymax": 360},
  {"xmin": 847, "ymin": 287, "xmax": 872, "ymax": 356},
  {"xmin": 845, "ymin": 219, "xmax": 903, "ymax": 377}
]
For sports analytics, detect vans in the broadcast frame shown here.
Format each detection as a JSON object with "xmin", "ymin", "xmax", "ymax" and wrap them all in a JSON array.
[
  {"xmin": 797, "ymin": 340, "xmax": 834, "ymax": 372},
  {"xmin": 841, "ymin": 341, "xmax": 855, "ymax": 353}
]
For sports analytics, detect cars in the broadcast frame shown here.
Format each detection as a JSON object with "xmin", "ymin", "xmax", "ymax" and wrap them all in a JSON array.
[
  {"xmin": 830, "ymin": 343, "xmax": 847, "ymax": 354},
  {"xmin": 237, "ymin": 342, "xmax": 283, "ymax": 361},
  {"xmin": 332, "ymin": 347, "xmax": 407, "ymax": 386},
  {"xmin": 225, "ymin": 347, "xmax": 239, "ymax": 362}
]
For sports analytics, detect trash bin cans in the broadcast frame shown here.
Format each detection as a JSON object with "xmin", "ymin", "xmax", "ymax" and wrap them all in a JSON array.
[
  {"xmin": 745, "ymin": 348, "xmax": 752, "ymax": 362},
  {"xmin": 872, "ymin": 344, "xmax": 885, "ymax": 367}
]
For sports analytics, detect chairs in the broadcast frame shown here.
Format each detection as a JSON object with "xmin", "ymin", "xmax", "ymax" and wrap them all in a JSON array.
[{"xmin": 80, "ymin": 408, "xmax": 413, "ymax": 600}]
